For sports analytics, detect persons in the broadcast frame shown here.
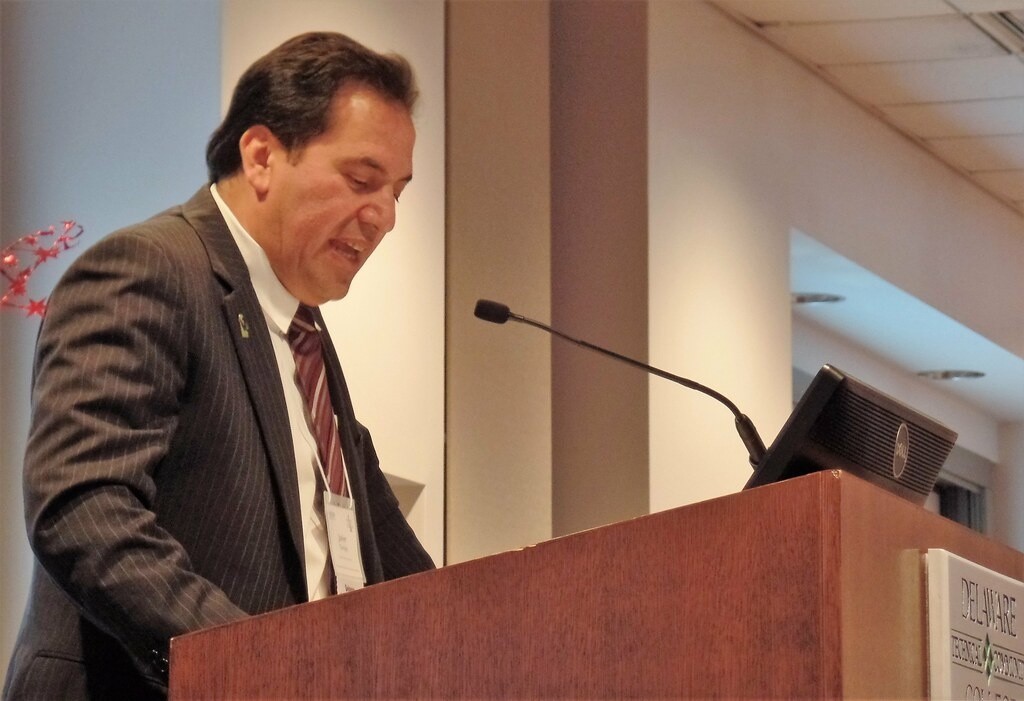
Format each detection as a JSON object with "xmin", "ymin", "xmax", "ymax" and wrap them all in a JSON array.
[{"xmin": 0, "ymin": 31, "xmax": 439, "ymax": 701}]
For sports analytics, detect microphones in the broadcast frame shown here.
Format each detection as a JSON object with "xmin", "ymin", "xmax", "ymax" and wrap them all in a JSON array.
[{"xmin": 474, "ymin": 300, "xmax": 767, "ymax": 469}]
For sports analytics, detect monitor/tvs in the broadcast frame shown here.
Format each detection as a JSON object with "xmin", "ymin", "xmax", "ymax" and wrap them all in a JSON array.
[{"xmin": 743, "ymin": 364, "xmax": 958, "ymax": 491}]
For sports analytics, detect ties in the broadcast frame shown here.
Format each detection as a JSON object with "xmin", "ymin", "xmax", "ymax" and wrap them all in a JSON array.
[{"xmin": 286, "ymin": 305, "xmax": 352, "ymax": 590}]
